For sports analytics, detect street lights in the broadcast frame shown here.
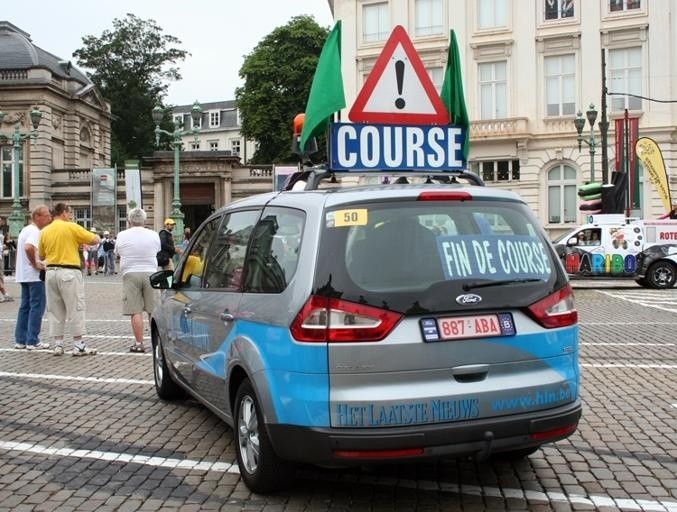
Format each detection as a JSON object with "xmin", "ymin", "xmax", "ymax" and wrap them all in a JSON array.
[
  {"xmin": 0, "ymin": 101, "xmax": 42, "ymax": 244},
  {"xmin": 572, "ymin": 102, "xmax": 602, "ymax": 180},
  {"xmin": 150, "ymin": 97, "xmax": 206, "ymax": 274}
]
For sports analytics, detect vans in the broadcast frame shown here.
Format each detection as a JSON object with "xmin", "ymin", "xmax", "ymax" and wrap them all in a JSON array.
[{"xmin": 148, "ymin": 164, "xmax": 584, "ymax": 497}]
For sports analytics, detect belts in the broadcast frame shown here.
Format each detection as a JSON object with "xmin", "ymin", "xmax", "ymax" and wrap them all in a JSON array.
[{"xmin": 46, "ymin": 264, "xmax": 80, "ymax": 270}]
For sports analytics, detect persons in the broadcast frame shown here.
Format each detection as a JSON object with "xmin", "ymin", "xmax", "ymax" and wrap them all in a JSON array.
[
  {"xmin": 37, "ymin": 202, "xmax": 101, "ymax": 357},
  {"xmin": 113, "ymin": 208, "xmax": 162, "ymax": 353},
  {"xmin": 589, "ymin": 232, "xmax": 600, "ymax": 246},
  {"xmin": 578, "ymin": 232, "xmax": 587, "ymax": 246},
  {"xmin": 82, "ymin": 226, "xmax": 118, "ymax": 276},
  {"xmin": 0, "ymin": 214, "xmax": 15, "ymax": 303},
  {"xmin": 158, "ymin": 218, "xmax": 203, "ymax": 284},
  {"xmin": 13, "ymin": 205, "xmax": 53, "ymax": 349}
]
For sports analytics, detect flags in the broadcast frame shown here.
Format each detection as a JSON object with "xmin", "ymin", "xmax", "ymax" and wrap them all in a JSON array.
[
  {"xmin": 299, "ymin": 20, "xmax": 346, "ymax": 155},
  {"xmin": 438, "ymin": 28, "xmax": 471, "ymax": 163}
]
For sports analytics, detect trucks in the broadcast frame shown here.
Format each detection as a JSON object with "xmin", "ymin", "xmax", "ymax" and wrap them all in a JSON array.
[{"xmin": 552, "ymin": 213, "xmax": 677, "ymax": 289}]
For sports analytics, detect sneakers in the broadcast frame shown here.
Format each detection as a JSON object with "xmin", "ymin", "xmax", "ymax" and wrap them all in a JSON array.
[
  {"xmin": 54, "ymin": 345, "xmax": 64, "ymax": 355},
  {"xmin": 72, "ymin": 345, "xmax": 97, "ymax": 356},
  {"xmin": 130, "ymin": 343, "xmax": 145, "ymax": 352},
  {"xmin": 4, "ymin": 295, "xmax": 13, "ymax": 301},
  {"xmin": 87, "ymin": 271, "xmax": 117, "ymax": 276},
  {"xmin": 15, "ymin": 343, "xmax": 26, "ymax": 349},
  {"xmin": 25, "ymin": 341, "xmax": 49, "ymax": 350}
]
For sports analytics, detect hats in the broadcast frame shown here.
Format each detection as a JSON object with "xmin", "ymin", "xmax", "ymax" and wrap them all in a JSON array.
[
  {"xmin": 164, "ymin": 219, "xmax": 176, "ymax": 225},
  {"xmin": 90, "ymin": 228, "xmax": 98, "ymax": 233}
]
